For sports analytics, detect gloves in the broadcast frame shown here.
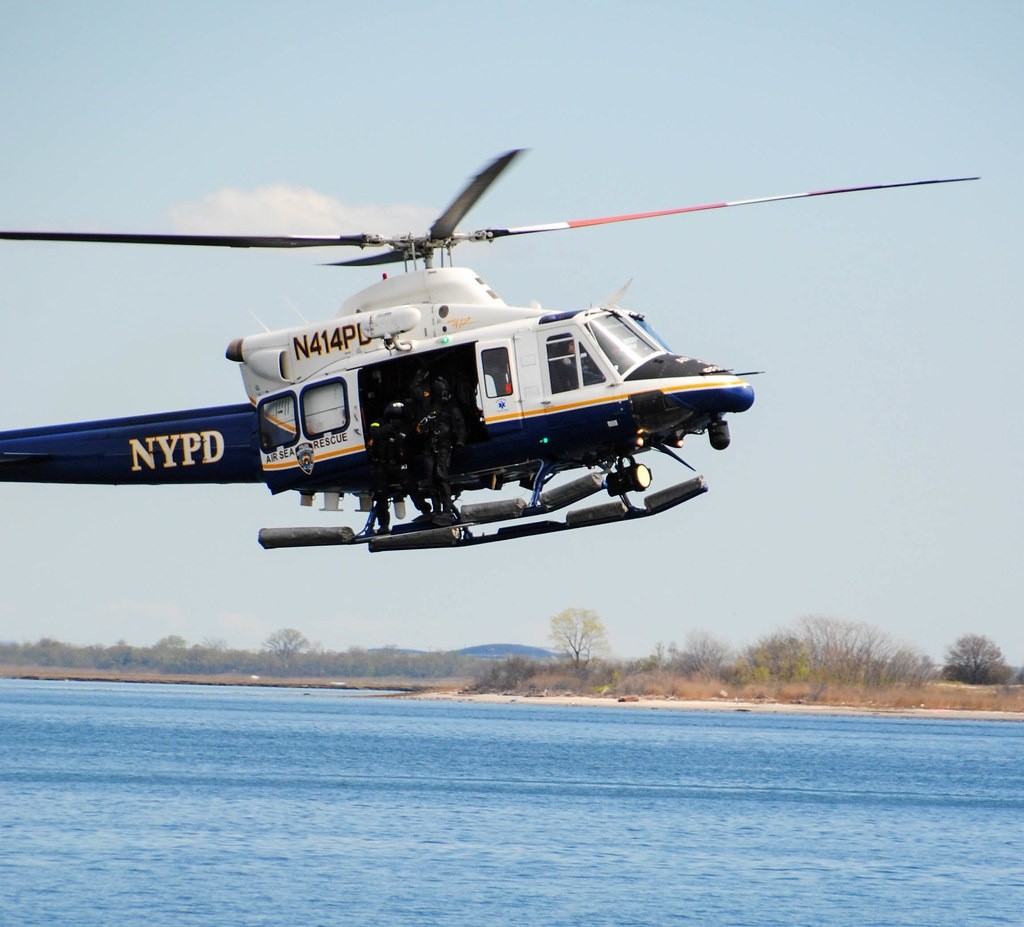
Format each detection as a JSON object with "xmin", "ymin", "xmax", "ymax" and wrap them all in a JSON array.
[{"xmin": 454, "ymin": 441, "xmax": 466, "ymax": 457}]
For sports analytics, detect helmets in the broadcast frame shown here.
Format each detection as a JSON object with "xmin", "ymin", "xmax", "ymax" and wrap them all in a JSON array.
[
  {"xmin": 383, "ymin": 399, "xmax": 416, "ymax": 424},
  {"xmin": 400, "ymin": 355, "xmax": 427, "ymax": 386}
]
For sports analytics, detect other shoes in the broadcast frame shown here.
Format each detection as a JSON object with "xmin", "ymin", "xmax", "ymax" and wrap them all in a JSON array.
[
  {"xmin": 412, "ymin": 511, "xmax": 434, "ymax": 523},
  {"xmin": 432, "ymin": 511, "xmax": 453, "ymax": 526},
  {"xmin": 376, "ymin": 526, "xmax": 390, "ymax": 535}
]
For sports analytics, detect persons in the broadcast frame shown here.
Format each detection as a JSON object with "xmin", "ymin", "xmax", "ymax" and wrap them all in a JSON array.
[
  {"xmin": 366, "ymin": 368, "xmax": 471, "ymax": 534},
  {"xmin": 548, "ymin": 337, "xmax": 606, "ymax": 394}
]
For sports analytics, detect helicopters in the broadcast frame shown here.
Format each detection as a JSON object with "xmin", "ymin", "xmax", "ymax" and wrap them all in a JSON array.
[{"xmin": 0, "ymin": 144, "xmax": 985, "ymax": 562}]
